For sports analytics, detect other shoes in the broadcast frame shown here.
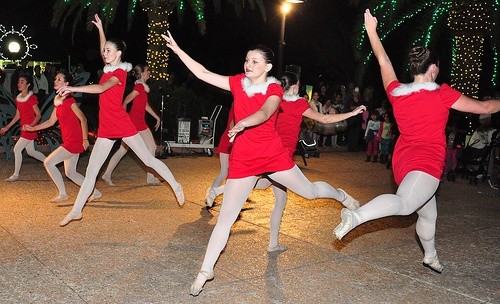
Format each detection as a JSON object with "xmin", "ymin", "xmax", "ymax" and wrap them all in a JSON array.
[
  {"xmin": 424, "ymin": 253, "xmax": 443, "ymax": 271},
  {"xmin": 50, "ymin": 195, "xmax": 69, "ymax": 203},
  {"xmin": 174, "ymin": 183, "xmax": 185, "ymax": 206},
  {"xmin": 207, "ymin": 188, "xmax": 217, "ymax": 207},
  {"xmin": 365, "ymin": 155, "xmax": 391, "ymax": 170},
  {"xmin": 267, "ymin": 244, "xmax": 288, "ymax": 251},
  {"xmin": 447, "ymin": 173, "xmax": 498, "ymax": 190},
  {"xmin": 5, "ymin": 175, "xmax": 19, "ymax": 181},
  {"xmin": 88, "ymin": 192, "xmax": 103, "ymax": 202},
  {"xmin": 333, "ymin": 208, "xmax": 354, "ymax": 240},
  {"xmin": 315, "ymin": 145, "xmax": 345, "ymax": 150},
  {"xmin": 147, "ymin": 176, "xmax": 164, "ymax": 185},
  {"xmin": 59, "ymin": 212, "xmax": 82, "ymax": 226},
  {"xmin": 102, "ymin": 174, "xmax": 118, "ymax": 187},
  {"xmin": 189, "ymin": 270, "xmax": 214, "ymax": 295},
  {"xmin": 337, "ymin": 188, "xmax": 360, "ymax": 210}
]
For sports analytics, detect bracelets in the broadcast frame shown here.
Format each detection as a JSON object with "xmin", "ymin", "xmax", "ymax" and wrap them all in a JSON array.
[{"xmin": 83, "ymin": 139, "xmax": 88, "ymax": 141}]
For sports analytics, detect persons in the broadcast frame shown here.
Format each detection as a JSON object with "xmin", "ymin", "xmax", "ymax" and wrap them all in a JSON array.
[
  {"xmin": 334, "ymin": 9, "xmax": 500, "ymax": 273},
  {"xmin": 439, "ymin": 94, "xmax": 500, "ymax": 191},
  {"xmin": 10, "ymin": 59, "xmax": 58, "ymax": 111},
  {"xmin": 206, "ymin": 72, "xmax": 366, "ymax": 252},
  {"xmin": 204, "ymin": 101, "xmax": 234, "ymax": 206},
  {"xmin": 23, "ymin": 71, "xmax": 102, "ymax": 203},
  {"xmin": 102, "ymin": 66, "xmax": 164, "ymax": 184},
  {"xmin": 161, "ymin": 30, "xmax": 358, "ymax": 296},
  {"xmin": 0, "ymin": 75, "xmax": 48, "ymax": 182},
  {"xmin": 59, "ymin": 14, "xmax": 185, "ymax": 225},
  {"xmin": 302, "ymin": 82, "xmax": 401, "ymax": 168}
]
